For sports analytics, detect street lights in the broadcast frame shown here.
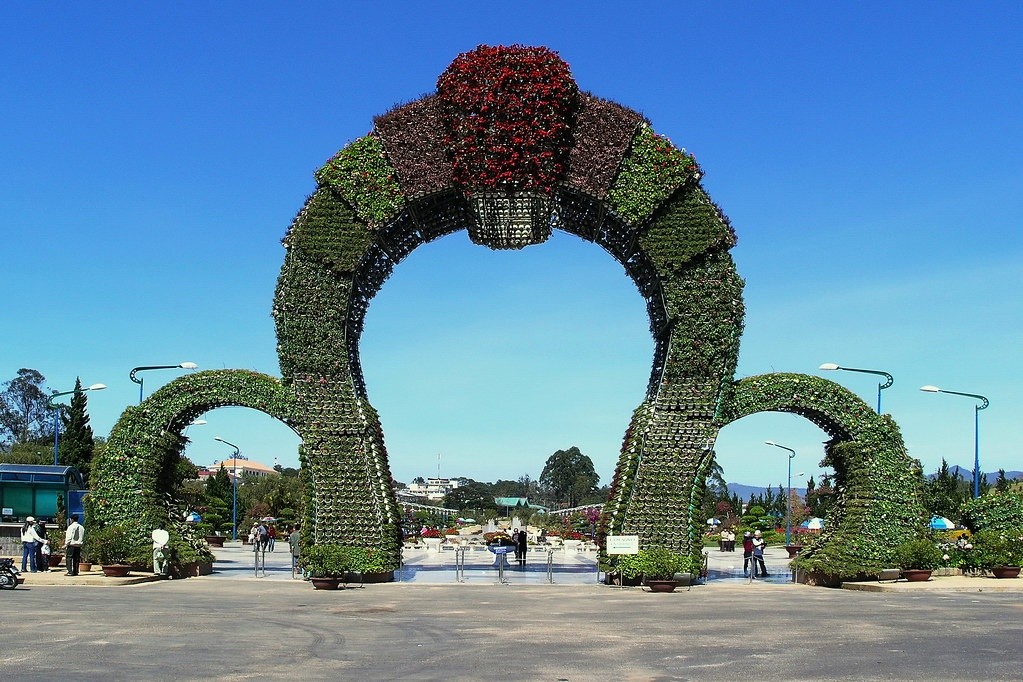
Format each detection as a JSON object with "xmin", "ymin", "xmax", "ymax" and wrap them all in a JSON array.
[
  {"xmin": 47, "ymin": 383, "xmax": 109, "ymax": 466},
  {"xmin": 818, "ymin": 362, "xmax": 895, "ymax": 416},
  {"xmin": 764, "ymin": 440, "xmax": 796, "ymax": 546},
  {"xmin": 130, "ymin": 361, "xmax": 199, "ymax": 405},
  {"xmin": 919, "ymin": 385, "xmax": 989, "ymax": 501},
  {"xmin": 214, "ymin": 436, "xmax": 240, "ymax": 540}
]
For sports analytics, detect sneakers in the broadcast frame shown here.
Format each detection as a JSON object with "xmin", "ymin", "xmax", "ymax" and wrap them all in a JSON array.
[{"xmin": 65, "ymin": 572, "xmax": 75, "ymax": 576}]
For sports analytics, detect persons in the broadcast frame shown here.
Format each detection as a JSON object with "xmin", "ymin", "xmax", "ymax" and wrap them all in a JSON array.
[
  {"xmin": 251, "ymin": 522, "xmax": 276, "ymax": 552},
  {"xmin": 63, "ymin": 515, "xmax": 85, "ymax": 576},
  {"xmin": 289, "ymin": 524, "xmax": 303, "ymax": 573},
  {"xmin": 721, "ymin": 527, "xmax": 736, "ymax": 551},
  {"xmin": 421, "ymin": 525, "xmax": 427, "ymax": 534},
  {"xmin": 152, "ymin": 522, "xmax": 170, "ymax": 576},
  {"xmin": 503, "ymin": 526, "xmax": 528, "ymax": 567},
  {"xmin": 743, "ymin": 530, "xmax": 770, "ymax": 578},
  {"xmin": 20, "ymin": 515, "xmax": 53, "ymax": 572}
]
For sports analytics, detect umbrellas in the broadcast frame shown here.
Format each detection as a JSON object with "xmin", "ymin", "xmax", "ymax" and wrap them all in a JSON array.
[
  {"xmin": 183, "ymin": 510, "xmax": 202, "ymax": 522},
  {"xmin": 930, "ymin": 514, "xmax": 954, "ymax": 530},
  {"xmin": 802, "ymin": 517, "xmax": 824, "ymax": 529},
  {"xmin": 707, "ymin": 517, "xmax": 721, "ymax": 524},
  {"xmin": 262, "ymin": 517, "xmax": 277, "ymax": 521}
]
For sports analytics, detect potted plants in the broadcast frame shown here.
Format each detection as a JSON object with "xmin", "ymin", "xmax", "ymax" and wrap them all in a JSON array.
[
  {"xmin": 969, "ymin": 529, "xmax": 1023, "ymax": 579},
  {"xmin": 306, "ymin": 544, "xmax": 352, "ymax": 588},
  {"xmin": 45, "ymin": 533, "xmax": 65, "ymax": 565},
  {"xmin": 94, "ymin": 524, "xmax": 132, "ymax": 577},
  {"xmin": 637, "ymin": 547, "xmax": 687, "ymax": 593},
  {"xmin": 895, "ymin": 538, "xmax": 944, "ymax": 582},
  {"xmin": 78, "ymin": 541, "xmax": 92, "ymax": 571}
]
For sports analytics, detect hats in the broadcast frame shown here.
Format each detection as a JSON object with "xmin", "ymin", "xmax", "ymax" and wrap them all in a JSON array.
[{"xmin": 26, "ymin": 516, "xmax": 36, "ymax": 522}]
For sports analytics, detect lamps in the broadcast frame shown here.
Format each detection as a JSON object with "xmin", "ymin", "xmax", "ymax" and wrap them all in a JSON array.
[{"xmin": 878, "ymin": 569, "xmax": 900, "ymax": 585}]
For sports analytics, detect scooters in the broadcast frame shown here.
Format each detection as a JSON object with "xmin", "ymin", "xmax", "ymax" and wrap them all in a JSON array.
[{"xmin": 0, "ymin": 545, "xmax": 21, "ymax": 591}]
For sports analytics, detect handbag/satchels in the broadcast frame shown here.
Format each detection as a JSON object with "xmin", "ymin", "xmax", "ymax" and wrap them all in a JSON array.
[
  {"xmin": 155, "ymin": 548, "xmax": 165, "ymax": 561},
  {"xmin": 41, "ymin": 541, "xmax": 51, "ymax": 556}
]
